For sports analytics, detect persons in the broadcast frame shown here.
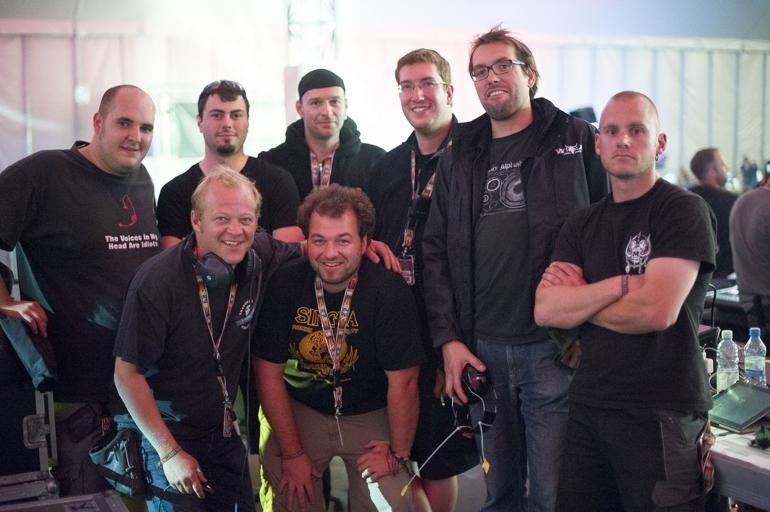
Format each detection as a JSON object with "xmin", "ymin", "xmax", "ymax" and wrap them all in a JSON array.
[
  {"xmin": 729, "ymin": 174, "xmax": 770, "ymax": 354},
  {"xmin": 687, "ymin": 146, "xmax": 734, "ymax": 291},
  {"xmin": 535, "ymin": 86, "xmax": 725, "ymax": 511},
  {"xmin": 114, "ymin": 163, "xmax": 401, "ymax": 512},
  {"xmin": 421, "ymin": 21, "xmax": 614, "ymax": 512},
  {"xmin": 372, "ymin": 50, "xmax": 483, "ymax": 511},
  {"xmin": 252, "ymin": 182, "xmax": 426, "ymax": 512},
  {"xmin": 157, "ymin": 80, "xmax": 305, "ymax": 250},
  {"xmin": 261, "ymin": 69, "xmax": 385, "ymax": 191},
  {"xmin": 1, "ymin": 85, "xmax": 157, "ymax": 512}
]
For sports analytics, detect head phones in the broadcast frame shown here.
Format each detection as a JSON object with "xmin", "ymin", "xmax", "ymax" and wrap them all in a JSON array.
[
  {"xmin": 184, "ymin": 231, "xmax": 261, "ymax": 289},
  {"xmin": 452, "ymin": 366, "xmax": 498, "ymax": 433}
]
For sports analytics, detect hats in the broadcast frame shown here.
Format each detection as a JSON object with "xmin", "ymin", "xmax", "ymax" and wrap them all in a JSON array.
[{"xmin": 298, "ymin": 69, "xmax": 344, "ymax": 99}]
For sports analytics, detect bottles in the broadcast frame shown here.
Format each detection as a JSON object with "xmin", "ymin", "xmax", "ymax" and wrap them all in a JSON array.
[
  {"xmin": 715, "ymin": 329, "xmax": 741, "ymax": 395},
  {"xmin": 744, "ymin": 326, "xmax": 768, "ymax": 389}
]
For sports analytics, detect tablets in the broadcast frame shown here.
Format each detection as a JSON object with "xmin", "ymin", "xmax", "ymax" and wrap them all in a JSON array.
[{"xmin": 708, "ymin": 380, "xmax": 770, "ymax": 433}]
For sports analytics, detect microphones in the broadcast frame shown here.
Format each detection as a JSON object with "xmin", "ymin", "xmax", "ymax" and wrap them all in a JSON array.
[{"xmin": 234, "ymin": 272, "xmax": 262, "ymax": 328}]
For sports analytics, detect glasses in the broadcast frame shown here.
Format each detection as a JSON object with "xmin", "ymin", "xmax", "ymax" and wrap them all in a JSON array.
[
  {"xmin": 470, "ymin": 58, "xmax": 526, "ymax": 80},
  {"xmin": 396, "ymin": 79, "xmax": 449, "ymax": 94},
  {"xmin": 200, "ymin": 80, "xmax": 246, "ymax": 99}
]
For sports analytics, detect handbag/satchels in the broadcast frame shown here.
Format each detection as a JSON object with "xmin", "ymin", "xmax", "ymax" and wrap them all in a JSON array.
[{"xmin": 87, "ymin": 431, "xmax": 145, "ymax": 500}]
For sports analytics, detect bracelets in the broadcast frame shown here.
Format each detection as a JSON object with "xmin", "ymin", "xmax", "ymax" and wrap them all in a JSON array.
[
  {"xmin": 161, "ymin": 445, "xmax": 180, "ymax": 464},
  {"xmin": 621, "ymin": 275, "xmax": 628, "ymax": 295},
  {"xmin": 388, "ymin": 443, "xmax": 410, "ymax": 462},
  {"xmin": 280, "ymin": 450, "xmax": 304, "ymax": 460}
]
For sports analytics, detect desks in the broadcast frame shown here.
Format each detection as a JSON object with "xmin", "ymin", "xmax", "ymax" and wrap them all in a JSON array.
[
  {"xmin": 703, "ymin": 284, "xmax": 748, "ymax": 329},
  {"xmin": 711, "ymin": 426, "xmax": 770, "ymax": 512}
]
